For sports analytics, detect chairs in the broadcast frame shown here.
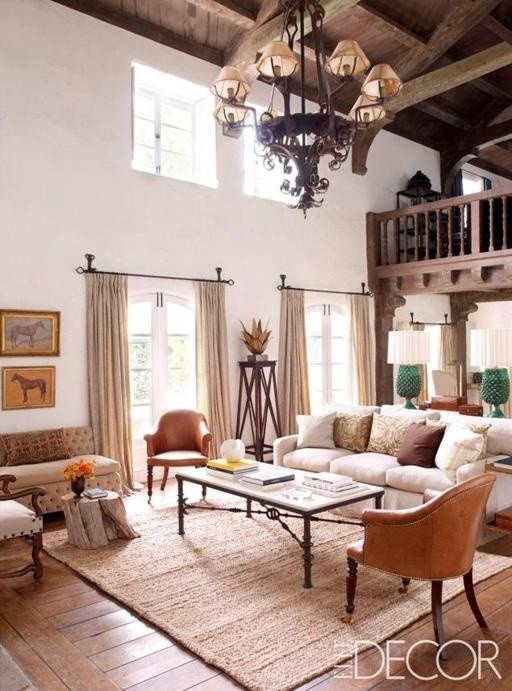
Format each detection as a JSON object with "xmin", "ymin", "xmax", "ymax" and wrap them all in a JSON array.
[
  {"xmin": 341, "ymin": 473, "xmax": 499, "ymax": 662},
  {"xmin": 142, "ymin": 407, "xmax": 214, "ymax": 503},
  {"xmin": 0, "ymin": 474, "xmax": 48, "ymax": 580}
]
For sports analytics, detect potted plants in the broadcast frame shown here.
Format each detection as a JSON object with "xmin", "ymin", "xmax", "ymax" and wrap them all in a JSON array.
[{"xmin": 236, "ymin": 315, "xmax": 275, "ymax": 364}]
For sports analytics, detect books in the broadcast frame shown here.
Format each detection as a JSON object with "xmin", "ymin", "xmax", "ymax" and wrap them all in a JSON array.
[
  {"xmin": 302, "ymin": 481, "xmax": 359, "ymax": 492},
  {"xmin": 303, "ymin": 472, "xmax": 354, "ymax": 485},
  {"xmin": 493, "ymin": 457, "xmax": 512, "ymax": 471},
  {"xmin": 238, "ymin": 478, "xmax": 295, "ymax": 491},
  {"xmin": 295, "ymin": 483, "xmax": 369, "ymax": 498},
  {"xmin": 242, "ymin": 467, "xmax": 296, "ymax": 485},
  {"xmin": 83, "ymin": 488, "xmax": 109, "ymax": 499},
  {"xmin": 206, "ymin": 465, "xmax": 259, "ymax": 474},
  {"xmin": 207, "ymin": 468, "xmax": 260, "ymax": 480},
  {"xmin": 205, "ymin": 458, "xmax": 259, "ymax": 471}
]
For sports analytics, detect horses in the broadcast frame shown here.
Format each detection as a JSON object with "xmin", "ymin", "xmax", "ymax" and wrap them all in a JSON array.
[
  {"xmin": 10, "ymin": 372, "xmax": 46, "ymax": 403},
  {"xmin": 10, "ymin": 319, "xmax": 45, "ymax": 349}
]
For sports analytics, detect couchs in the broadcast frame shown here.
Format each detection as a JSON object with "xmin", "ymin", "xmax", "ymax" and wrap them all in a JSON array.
[
  {"xmin": 272, "ymin": 400, "xmax": 512, "ymax": 523},
  {"xmin": 0, "ymin": 427, "xmax": 122, "ymax": 517}
]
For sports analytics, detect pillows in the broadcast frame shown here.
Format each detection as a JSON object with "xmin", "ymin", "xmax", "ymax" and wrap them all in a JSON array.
[
  {"xmin": 295, "ymin": 411, "xmax": 336, "ymax": 450},
  {"xmin": 333, "ymin": 412, "xmax": 373, "ymax": 454},
  {"xmin": 365, "ymin": 412, "xmax": 427, "ymax": 452},
  {"xmin": 435, "ymin": 419, "xmax": 492, "ymax": 485},
  {"xmin": 1, "ymin": 427, "xmax": 72, "ymax": 465},
  {"xmin": 396, "ymin": 423, "xmax": 445, "ymax": 468}
]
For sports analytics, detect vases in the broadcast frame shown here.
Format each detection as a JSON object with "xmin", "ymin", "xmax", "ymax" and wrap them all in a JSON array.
[{"xmin": 71, "ymin": 477, "xmax": 85, "ymax": 499}]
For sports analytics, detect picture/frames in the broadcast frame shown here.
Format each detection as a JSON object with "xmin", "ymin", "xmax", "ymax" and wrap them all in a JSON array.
[
  {"xmin": 1, "ymin": 310, "xmax": 60, "ymax": 357},
  {"xmin": 1, "ymin": 367, "xmax": 56, "ymax": 411}
]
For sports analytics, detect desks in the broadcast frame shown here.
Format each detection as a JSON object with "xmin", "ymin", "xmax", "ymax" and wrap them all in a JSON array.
[
  {"xmin": 60, "ymin": 488, "xmax": 142, "ymax": 550},
  {"xmin": 235, "ymin": 360, "xmax": 283, "ymax": 461}
]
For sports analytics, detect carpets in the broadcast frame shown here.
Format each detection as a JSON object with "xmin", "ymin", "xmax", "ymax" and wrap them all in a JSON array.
[{"xmin": 41, "ymin": 493, "xmax": 511, "ymax": 691}]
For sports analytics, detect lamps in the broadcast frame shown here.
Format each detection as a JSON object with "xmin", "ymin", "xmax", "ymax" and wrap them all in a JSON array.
[
  {"xmin": 387, "ymin": 330, "xmax": 430, "ymax": 409},
  {"xmin": 205, "ymin": 0, "xmax": 405, "ymax": 216},
  {"xmin": 470, "ymin": 327, "xmax": 512, "ymax": 418}
]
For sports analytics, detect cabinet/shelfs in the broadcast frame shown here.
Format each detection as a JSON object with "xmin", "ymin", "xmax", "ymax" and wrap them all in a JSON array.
[{"xmin": 395, "ymin": 169, "xmax": 460, "ymax": 263}]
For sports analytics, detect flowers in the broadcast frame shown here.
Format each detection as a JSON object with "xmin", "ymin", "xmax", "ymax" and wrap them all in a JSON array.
[{"xmin": 64, "ymin": 459, "xmax": 97, "ymax": 483}]
